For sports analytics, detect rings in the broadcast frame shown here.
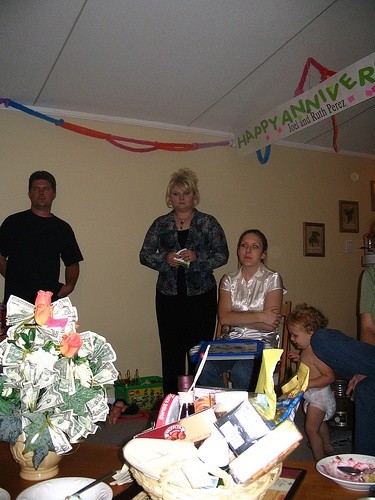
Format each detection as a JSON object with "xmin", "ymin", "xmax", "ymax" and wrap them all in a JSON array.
[
  {"xmin": 188, "ymin": 259, "xmax": 190, "ymax": 260},
  {"xmin": 187, "ymin": 253, "xmax": 188, "ymax": 256}
]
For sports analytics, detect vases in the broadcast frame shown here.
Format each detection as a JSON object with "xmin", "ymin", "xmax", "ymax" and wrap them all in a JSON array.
[{"xmin": 10, "ymin": 426, "xmax": 65, "ymax": 481}]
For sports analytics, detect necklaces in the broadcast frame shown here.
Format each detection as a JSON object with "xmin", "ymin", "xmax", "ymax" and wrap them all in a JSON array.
[{"xmin": 174, "ymin": 211, "xmax": 193, "ymax": 229}]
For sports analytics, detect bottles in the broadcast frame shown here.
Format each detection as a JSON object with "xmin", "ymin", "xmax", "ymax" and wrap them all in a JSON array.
[{"xmin": 177, "ymin": 375, "xmax": 195, "ymax": 419}]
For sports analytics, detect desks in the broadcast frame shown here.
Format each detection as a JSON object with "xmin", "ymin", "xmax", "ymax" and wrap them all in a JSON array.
[{"xmin": 2, "ymin": 441, "xmax": 371, "ymax": 500}]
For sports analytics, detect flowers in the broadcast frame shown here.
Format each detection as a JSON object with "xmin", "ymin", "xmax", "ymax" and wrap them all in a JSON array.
[{"xmin": 0, "ymin": 290, "xmax": 118, "ymax": 470}]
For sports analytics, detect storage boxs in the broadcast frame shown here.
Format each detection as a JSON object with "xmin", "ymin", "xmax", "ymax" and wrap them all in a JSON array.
[{"xmin": 114, "ymin": 376, "xmax": 164, "ymax": 407}]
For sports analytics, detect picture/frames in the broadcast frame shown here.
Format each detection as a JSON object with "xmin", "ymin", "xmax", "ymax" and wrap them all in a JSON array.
[
  {"xmin": 339, "ymin": 201, "xmax": 359, "ymax": 233},
  {"xmin": 303, "ymin": 222, "xmax": 325, "ymax": 257}
]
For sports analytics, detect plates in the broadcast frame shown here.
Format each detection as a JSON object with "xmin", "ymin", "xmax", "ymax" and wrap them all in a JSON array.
[
  {"xmin": 16, "ymin": 476, "xmax": 113, "ymax": 500},
  {"xmin": 0, "ymin": 488, "xmax": 11, "ymax": 500},
  {"xmin": 315, "ymin": 453, "xmax": 375, "ymax": 492}
]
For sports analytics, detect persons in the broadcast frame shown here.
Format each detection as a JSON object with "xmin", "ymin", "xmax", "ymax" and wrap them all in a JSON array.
[
  {"xmin": 140, "ymin": 168, "xmax": 229, "ymax": 419},
  {"xmin": 198, "ymin": 229, "xmax": 289, "ymax": 391},
  {"xmin": 0, "ymin": 171, "xmax": 84, "ymax": 305},
  {"xmin": 285, "ymin": 264, "xmax": 375, "ymax": 461}
]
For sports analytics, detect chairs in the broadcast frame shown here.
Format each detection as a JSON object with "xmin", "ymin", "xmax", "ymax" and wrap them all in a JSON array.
[{"xmin": 217, "ymin": 301, "xmax": 291, "ymax": 391}]
